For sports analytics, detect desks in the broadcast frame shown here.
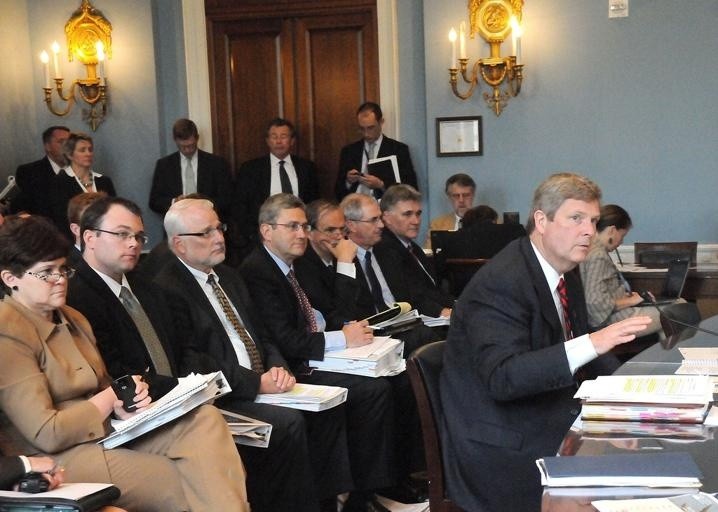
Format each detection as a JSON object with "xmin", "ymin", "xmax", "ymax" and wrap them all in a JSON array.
[
  {"xmin": 540, "ymin": 315, "xmax": 718, "ymax": 512},
  {"xmin": 617, "ymin": 262, "xmax": 718, "ymax": 320}
]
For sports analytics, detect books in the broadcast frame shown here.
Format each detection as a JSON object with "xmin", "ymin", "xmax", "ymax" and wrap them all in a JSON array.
[
  {"xmin": 367, "ymin": 154, "xmax": 402, "ymax": 198},
  {"xmin": 538, "ymin": 453, "xmax": 703, "ymax": 492},
  {"xmin": 582, "ymin": 422, "xmax": 704, "ymax": 437},
  {"xmin": 310, "ymin": 335, "xmax": 408, "ymax": 379},
  {"xmin": 253, "ymin": 381, "xmax": 350, "ymax": 413},
  {"xmin": 580, "ymin": 381, "xmax": 713, "ymax": 422}
]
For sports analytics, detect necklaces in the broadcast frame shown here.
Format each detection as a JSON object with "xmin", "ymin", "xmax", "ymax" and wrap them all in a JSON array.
[{"xmin": 76, "ymin": 171, "xmax": 93, "ymax": 187}]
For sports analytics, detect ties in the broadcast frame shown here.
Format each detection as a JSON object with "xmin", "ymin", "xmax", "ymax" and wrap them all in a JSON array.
[
  {"xmin": 361, "ymin": 143, "xmax": 376, "ymax": 196},
  {"xmin": 119, "ymin": 285, "xmax": 174, "ymax": 377},
  {"xmin": 184, "ymin": 158, "xmax": 196, "ymax": 195},
  {"xmin": 556, "ymin": 278, "xmax": 586, "ymax": 387},
  {"xmin": 278, "ymin": 161, "xmax": 293, "ymax": 194},
  {"xmin": 287, "ymin": 269, "xmax": 318, "ymax": 333},
  {"xmin": 206, "ymin": 273, "xmax": 265, "ymax": 376},
  {"xmin": 365, "ymin": 251, "xmax": 391, "ymax": 312}
]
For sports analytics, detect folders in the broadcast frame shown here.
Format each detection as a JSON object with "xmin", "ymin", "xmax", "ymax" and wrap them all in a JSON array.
[{"xmin": 96, "ymin": 370, "xmax": 232, "ymax": 450}]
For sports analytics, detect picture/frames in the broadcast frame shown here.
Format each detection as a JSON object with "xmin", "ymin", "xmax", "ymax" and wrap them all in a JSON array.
[{"xmin": 436, "ymin": 116, "xmax": 482, "ymax": 157}]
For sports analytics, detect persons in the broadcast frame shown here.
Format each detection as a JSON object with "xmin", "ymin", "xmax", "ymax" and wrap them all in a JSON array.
[
  {"xmin": 306, "ymin": 202, "xmax": 374, "ymax": 326},
  {"xmin": 334, "ymin": 101, "xmax": 420, "ymax": 196},
  {"xmin": 240, "ymin": 119, "xmax": 320, "ymax": 219},
  {"xmin": 0, "ymin": 214, "xmax": 252, "ymax": 512},
  {"xmin": 1, "ymin": 455, "xmax": 127, "ymax": 511},
  {"xmin": 424, "ymin": 174, "xmax": 476, "ymax": 255},
  {"xmin": 145, "ymin": 120, "xmax": 232, "ymax": 226},
  {"xmin": 440, "ymin": 174, "xmax": 652, "ymax": 512},
  {"xmin": 65, "ymin": 199, "xmax": 289, "ymax": 512},
  {"xmin": 50, "ymin": 134, "xmax": 120, "ymax": 217},
  {"xmin": 238, "ymin": 193, "xmax": 428, "ymax": 511},
  {"xmin": 580, "ymin": 205, "xmax": 688, "ymax": 340},
  {"xmin": 13, "ymin": 126, "xmax": 76, "ymax": 231},
  {"xmin": 136, "ymin": 197, "xmax": 368, "ymax": 511},
  {"xmin": 380, "ymin": 184, "xmax": 453, "ymax": 330},
  {"xmin": 460, "ymin": 206, "xmax": 500, "ymax": 231},
  {"xmin": 342, "ymin": 194, "xmax": 424, "ymax": 353}
]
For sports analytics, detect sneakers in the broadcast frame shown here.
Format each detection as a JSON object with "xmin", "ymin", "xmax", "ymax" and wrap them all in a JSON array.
[
  {"xmin": 342, "ymin": 494, "xmax": 392, "ymax": 512},
  {"xmin": 376, "ymin": 475, "xmax": 427, "ymax": 504}
]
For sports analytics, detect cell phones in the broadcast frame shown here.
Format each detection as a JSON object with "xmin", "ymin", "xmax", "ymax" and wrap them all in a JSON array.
[
  {"xmin": 19, "ymin": 474, "xmax": 48, "ymax": 494},
  {"xmin": 110, "ymin": 374, "xmax": 137, "ymax": 413},
  {"xmin": 355, "ymin": 172, "xmax": 364, "ymax": 176}
]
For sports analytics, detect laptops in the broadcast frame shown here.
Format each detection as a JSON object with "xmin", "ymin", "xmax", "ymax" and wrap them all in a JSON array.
[{"xmin": 641, "ymin": 258, "xmax": 691, "ymax": 305}]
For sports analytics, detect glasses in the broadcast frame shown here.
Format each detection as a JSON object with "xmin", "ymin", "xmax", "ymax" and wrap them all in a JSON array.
[
  {"xmin": 451, "ymin": 192, "xmax": 473, "ymax": 199},
  {"xmin": 178, "ymin": 222, "xmax": 228, "ymax": 238},
  {"xmin": 91, "ymin": 227, "xmax": 150, "ymax": 246},
  {"xmin": 349, "ymin": 214, "xmax": 388, "ymax": 224},
  {"xmin": 24, "ymin": 265, "xmax": 77, "ymax": 283},
  {"xmin": 270, "ymin": 221, "xmax": 312, "ymax": 232},
  {"xmin": 313, "ymin": 224, "xmax": 350, "ymax": 239}
]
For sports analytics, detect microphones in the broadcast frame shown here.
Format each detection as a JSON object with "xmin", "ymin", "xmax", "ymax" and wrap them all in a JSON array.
[{"xmin": 638, "ymin": 285, "xmax": 718, "ymax": 336}]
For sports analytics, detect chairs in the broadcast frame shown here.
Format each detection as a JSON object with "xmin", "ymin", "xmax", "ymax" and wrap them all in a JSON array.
[
  {"xmin": 444, "ymin": 259, "xmax": 488, "ymax": 296},
  {"xmin": 406, "ymin": 340, "xmax": 460, "ymax": 512}
]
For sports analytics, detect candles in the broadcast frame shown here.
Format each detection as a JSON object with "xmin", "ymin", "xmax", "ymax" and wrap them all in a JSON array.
[
  {"xmin": 459, "ymin": 25, "xmax": 468, "ymax": 59},
  {"xmin": 516, "ymin": 26, "xmax": 522, "ymax": 65},
  {"xmin": 510, "ymin": 16, "xmax": 516, "ymax": 56},
  {"xmin": 449, "ymin": 27, "xmax": 457, "ymax": 68},
  {"xmin": 99, "ymin": 52, "xmax": 106, "ymax": 79},
  {"xmin": 41, "ymin": 50, "xmax": 50, "ymax": 88},
  {"xmin": 50, "ymin": 41, "xmax": 60, "ymax": 78}
]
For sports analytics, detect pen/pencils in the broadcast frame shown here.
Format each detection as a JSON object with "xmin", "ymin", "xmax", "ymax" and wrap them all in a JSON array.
[{"xmin": 344, "ymin": 322, "xmax": 385, "ymax": 330}]
